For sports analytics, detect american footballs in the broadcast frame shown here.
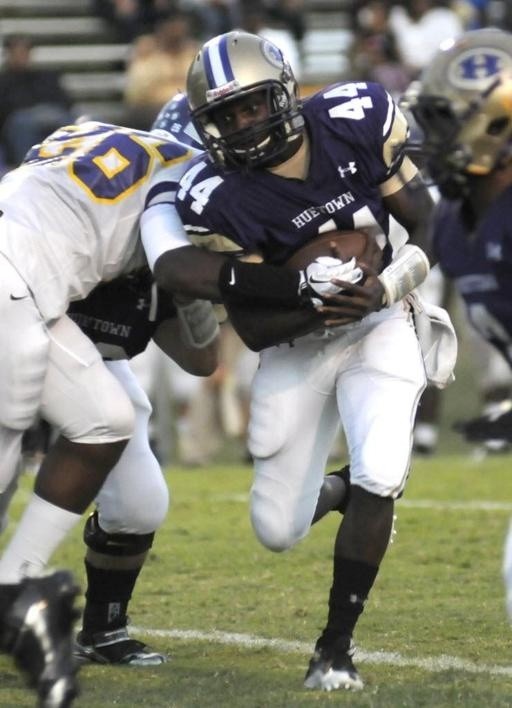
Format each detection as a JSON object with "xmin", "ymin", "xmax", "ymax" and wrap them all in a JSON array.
[{"xmin": 281, "ymin": 230, "xmax": 381, "ymax": 279}]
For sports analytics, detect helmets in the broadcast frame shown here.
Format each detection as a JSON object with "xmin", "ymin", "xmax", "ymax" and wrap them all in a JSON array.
[
  {"xmin": 188, "ymin": 29, "xmax": 305, "ymax": 167},
  {"xmin": 151, "ymin": 93, "xmax": 205, "ymax": 151},
  {"xmin": 392, "ymin": 27, "xmax": 512, "ymax": 191}
]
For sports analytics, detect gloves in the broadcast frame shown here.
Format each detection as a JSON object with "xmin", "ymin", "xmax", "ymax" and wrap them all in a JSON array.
[{"xmin": 297, "ymin": 257, "xmax": 361, "ymax": 310}]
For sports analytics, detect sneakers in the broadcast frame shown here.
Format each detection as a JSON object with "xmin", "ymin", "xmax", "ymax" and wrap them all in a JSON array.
[
  {"xmin": 306, "ymin": 646, "xmax": 365, "ymax": 691},
  {"xmin": 77, "ymin": 626, "xmax": 170, "ymax": 667},
  {"xmin": 453, "ymin": 402, "xmax": 511, "ymax": 440}
]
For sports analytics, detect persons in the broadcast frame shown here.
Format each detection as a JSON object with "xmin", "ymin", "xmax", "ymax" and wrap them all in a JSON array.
[
  {"xmin": 1, "ymin": 85, "xmax": 369, "ymax": 687},
  {"xmin": 2, "ymin": 0, "xmax": 512, "ymax": 471},
  {"xmin": 2, "ymin": 219, "xmax": 220, "ymax": 671},
  {"xmin": 173, "ymin": 26, "xmax": 451, "ymax": 694}
]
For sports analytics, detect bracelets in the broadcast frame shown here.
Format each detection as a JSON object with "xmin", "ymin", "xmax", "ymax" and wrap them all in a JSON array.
[{"xmin": 375, "ymin": 240, "xmax": 434, "ymax": 310}]
